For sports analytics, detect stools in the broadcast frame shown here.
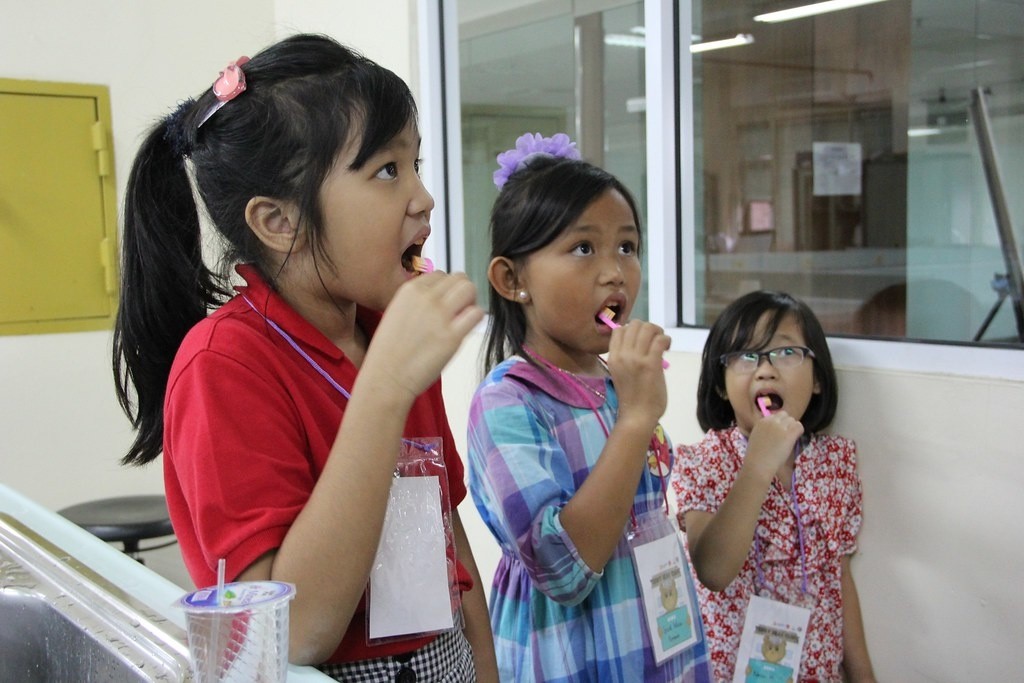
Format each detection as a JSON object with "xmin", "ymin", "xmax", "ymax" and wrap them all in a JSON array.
[{"xmin": 56, "ymin": 495, "xmax": 179, "ymax": 565}]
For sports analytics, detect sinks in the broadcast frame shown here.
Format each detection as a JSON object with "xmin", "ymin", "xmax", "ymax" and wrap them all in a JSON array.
[{"xmin": 1, "ymin": 590, "xmax": 155, "ymax": 683}]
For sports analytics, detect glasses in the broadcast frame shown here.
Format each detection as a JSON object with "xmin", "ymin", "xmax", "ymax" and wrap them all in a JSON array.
[{"xmin": 721, "ymin": 345, "xmax": 815, "ymax": 374}]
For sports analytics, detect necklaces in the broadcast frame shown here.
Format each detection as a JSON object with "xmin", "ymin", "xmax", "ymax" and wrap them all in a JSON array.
[{"xmin": 519, "ymin": 341, "xmax": 618, "ymax": 429}]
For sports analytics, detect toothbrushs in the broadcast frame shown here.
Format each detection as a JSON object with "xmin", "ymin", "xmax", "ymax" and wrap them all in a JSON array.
[
  {"xmin": 412, "ymin": 255, "xmax": 435, "ymax": 277},
  {"xmin": 599, "ymin": 307, "xmax": 669, "ymax": 370},
  {"xmin": 756, "ymin": 396, "xmax": 772, "ymax": 416}
]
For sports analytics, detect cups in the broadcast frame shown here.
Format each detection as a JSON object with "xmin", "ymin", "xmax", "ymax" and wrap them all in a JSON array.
[{"xmin": 179, "ymin": 580, "xmax": 295, "ymax": 683}]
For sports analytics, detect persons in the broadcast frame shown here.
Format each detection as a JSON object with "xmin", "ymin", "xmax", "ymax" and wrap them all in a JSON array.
[
  {"xmin": 466, "ymin": 134, "xmax": 709, "ymax": 683},
  {"xmin": 114, "ymin": 32, "xmax": 499, "ymax": 683},
  {"xmin": 672, "ymin": 292, "xmax": 879, "ymax": 683}
]
